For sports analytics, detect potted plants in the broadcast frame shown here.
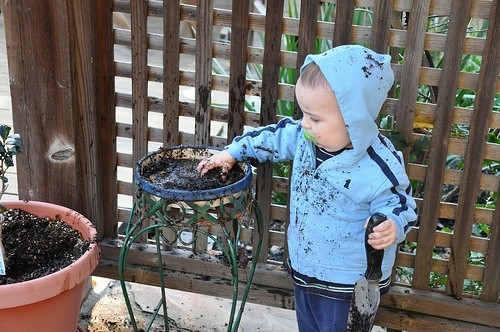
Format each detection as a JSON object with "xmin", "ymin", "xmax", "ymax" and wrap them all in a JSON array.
[{"xmin": 0, "ymin": 123, "xmax": 96, "ymax": 332}]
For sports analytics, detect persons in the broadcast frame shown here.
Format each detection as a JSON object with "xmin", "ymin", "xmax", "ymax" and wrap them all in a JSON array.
[{"xmin": 196, "ymin": 44, "xmax": 417, "ymax": 331}]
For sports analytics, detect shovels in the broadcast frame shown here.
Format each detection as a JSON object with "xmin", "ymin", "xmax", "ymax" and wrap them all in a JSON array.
[{"xmin": 346, "ymin": 212, "xmax": 388, "ymax": 332}]
